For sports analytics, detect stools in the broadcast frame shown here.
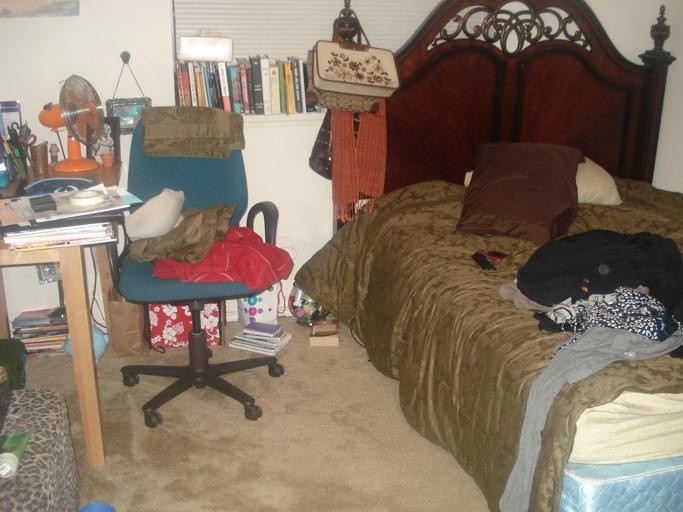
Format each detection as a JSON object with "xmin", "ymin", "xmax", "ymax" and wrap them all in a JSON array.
[{"xmin": 1, "ymin": 389, "xmax": 81, "ymax": 512}]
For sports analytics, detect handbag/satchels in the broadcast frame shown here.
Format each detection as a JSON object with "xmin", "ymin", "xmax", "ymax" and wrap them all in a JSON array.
[
  {"xmin": 305, "ymin": 40, "xmax": 400, "ymax": 113},
  {"xmin": 310, "ymin": 110, "xmax": 360, "ymax": 180},
  {"xmin": 106, "ymin": 285, "xmax": 146, "ymax": 359}
]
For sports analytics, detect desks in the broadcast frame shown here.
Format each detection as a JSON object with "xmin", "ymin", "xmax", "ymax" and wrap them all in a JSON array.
[{"xmin": 1, "ymin": 160, "xmax": 119, "ymax": 468}]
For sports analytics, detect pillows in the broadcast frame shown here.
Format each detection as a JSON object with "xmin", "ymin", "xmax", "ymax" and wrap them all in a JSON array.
[
  {"xmin": 453, "ymin": 142, "xmax": 584, "ymax": 246},
  {"xmin": 463, "ymin": 155, "xmax": 623, "ymax": 208}
]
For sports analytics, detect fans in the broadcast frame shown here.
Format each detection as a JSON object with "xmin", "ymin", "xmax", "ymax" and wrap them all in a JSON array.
[{"xmin": 39, "ymin": 74, "xmax": 104, "ymax": 173}]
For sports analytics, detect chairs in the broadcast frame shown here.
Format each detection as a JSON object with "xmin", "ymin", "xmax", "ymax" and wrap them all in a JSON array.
[{"xmin": 107, "ymin": 106, "xmax": 285, "ymax": 428}]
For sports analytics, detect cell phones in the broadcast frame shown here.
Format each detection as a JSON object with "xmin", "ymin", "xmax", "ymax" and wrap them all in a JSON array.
[{"xmin": 489, "ymin": 249, "xmax": 509, "ymax": 260}]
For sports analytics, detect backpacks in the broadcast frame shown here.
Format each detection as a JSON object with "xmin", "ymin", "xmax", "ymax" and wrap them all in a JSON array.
[{"xmin": 517, "ymin": 230, "xmax": 682, "ymax": 314}]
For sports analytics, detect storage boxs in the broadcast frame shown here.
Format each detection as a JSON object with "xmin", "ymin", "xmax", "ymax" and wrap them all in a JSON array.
[{"xmin": 148, "ymin": 301, "xmax": 221, "ymax": 348}]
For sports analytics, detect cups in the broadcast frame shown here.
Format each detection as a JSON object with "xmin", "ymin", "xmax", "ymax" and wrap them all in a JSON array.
[{"xmin": 30, "ymin": 141, "xmax": 50, "ymax": 176}]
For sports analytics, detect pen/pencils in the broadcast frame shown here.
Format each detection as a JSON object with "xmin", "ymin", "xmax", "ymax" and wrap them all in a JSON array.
[{"xmin": 0, "ymin": 126, "xmax": 35, "ymax": 182}]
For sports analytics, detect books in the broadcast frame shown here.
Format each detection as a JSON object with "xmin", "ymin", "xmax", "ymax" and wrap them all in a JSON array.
[
  {"xmin": 2, "ymin": 219, "xmax": 117, "ymax": 251},
  {"xmin": 175, "ymin": 53, "xmax": 322, "ymax": 116},
  {"xmin": 309, "ymin": 318, "xmax": 341, "ymax": 347},
  {"xmin": 10, "ymin": 309, "xmax": 68, "ymax": 354},
  {"xmin": 228, "ymin": 321, "xmax": 293, "ymax": 357}
]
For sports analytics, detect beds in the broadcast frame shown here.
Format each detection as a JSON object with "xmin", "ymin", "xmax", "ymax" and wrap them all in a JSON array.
[{"xmin": 332, "ymin": 1, "xmax": 683, "ymax": 510}]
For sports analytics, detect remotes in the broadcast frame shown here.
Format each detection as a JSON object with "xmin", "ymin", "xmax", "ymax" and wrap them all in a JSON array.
[{"xmin": 471, "ymin": 252, "xmax": 496, "ymax": 272}]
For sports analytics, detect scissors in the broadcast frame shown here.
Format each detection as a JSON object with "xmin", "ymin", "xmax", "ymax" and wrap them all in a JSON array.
[{"xmin": 11, "ymin": 122, "xmax": 37, "ymax": 146}]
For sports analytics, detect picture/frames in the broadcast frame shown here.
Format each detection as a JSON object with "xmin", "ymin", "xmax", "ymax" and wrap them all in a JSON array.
[{"xmin": 106, "ymin": 96, "xmax": 152, "ymax": 134}]
